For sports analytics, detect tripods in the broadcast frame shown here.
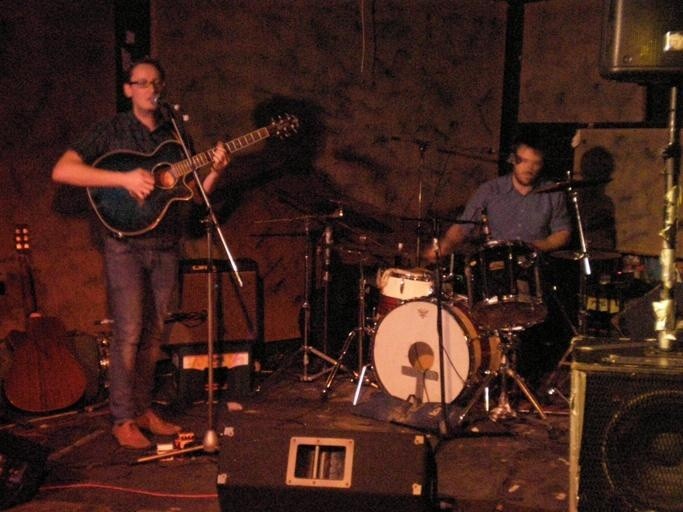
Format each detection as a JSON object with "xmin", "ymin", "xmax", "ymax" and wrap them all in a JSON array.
[
  {"xmin": 320, "ymin": 254, "xmax": 376, "ymax": 405},
  {"xmin": 526, "ymin": 186, "xmax": 644, "ymax": 416},
  {"xmin": 254, "ymin": 207, "xmax": 378, "ymax": 393},
  {"xmin": 131, "ymin": 123, "xmax": 245, "ymax": 465},
  {"xmin": 459, "ymin": 328, "xmax": 561, "ymax": 442}
]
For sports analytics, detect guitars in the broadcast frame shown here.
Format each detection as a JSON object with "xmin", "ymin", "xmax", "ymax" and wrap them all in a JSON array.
[
  {"xmin": 3, "ymin": 223, "xmax": 85, "ymax": 413},
  {"xmin": 87, "ymin": 113, "xmax": 299, "ymax": 238}
]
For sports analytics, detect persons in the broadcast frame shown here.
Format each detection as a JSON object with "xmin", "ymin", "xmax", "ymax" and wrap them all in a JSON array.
[
  {"xmin": 423, "ymin": 139, "xmax": 574, "ymax": 263},
  {"xmin": 49, "ymin": 62, "xmax": 239, "ymax": 453}
]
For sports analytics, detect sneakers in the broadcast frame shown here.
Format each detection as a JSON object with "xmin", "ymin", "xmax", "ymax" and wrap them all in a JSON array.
[
  {"xmin": 137, "ymin": 407, "xmax": 181, "ymax": 436},
  {"xmin": 112, "ymin": 419, "xmax": 152, "ymax": 450}
]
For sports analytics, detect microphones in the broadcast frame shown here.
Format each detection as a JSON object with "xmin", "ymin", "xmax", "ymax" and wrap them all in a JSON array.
[
  {"xmin": 151, "ymin": 95, "xmax": 190, "ymax": 120},
  {"xmin": 484, "ymin": 147, "xmax": 519, "ymax": 164}
]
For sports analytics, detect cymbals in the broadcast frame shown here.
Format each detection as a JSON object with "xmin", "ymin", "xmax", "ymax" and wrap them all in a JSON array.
[
  {"xmin": 549, "ymin": 250, "xmax": 621, "ymax": 261},
  {"xmin": 538, "ymin": 179, "xmax": 612, "ymax": 193},
  {"xmin": 317, "ymin": 191, "xmax": 381, "ymax": 213}
]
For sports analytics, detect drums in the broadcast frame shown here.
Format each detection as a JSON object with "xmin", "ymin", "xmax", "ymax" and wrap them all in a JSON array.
[
  {"xmin": 466, "ymin": 239, "xmax": 546, "ymax": 330},
  {"xmin": 381, "ymin": 269, "xmax": 436, "ymax": 300},
  {"xmin": 373, "ymin": 298, "xmax": 501, "ymax": 409}
]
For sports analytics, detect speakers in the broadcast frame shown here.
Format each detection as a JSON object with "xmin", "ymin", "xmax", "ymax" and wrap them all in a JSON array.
[
  {"xmin": 215, "ymin": 421, "xmax": 438, "ymax": 511},
  {"xmin": 567, "ymin": 342, "xmax": 683, "ymax": 512},
  {"xmin": 599, "ymin": 2, "xmax": 682, "ymax": 76}
]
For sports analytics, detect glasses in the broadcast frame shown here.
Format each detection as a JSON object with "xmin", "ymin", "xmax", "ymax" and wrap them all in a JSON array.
[{"xmin": 130, "ymin": 79, "xmax": 164, "ymax": 89}]
